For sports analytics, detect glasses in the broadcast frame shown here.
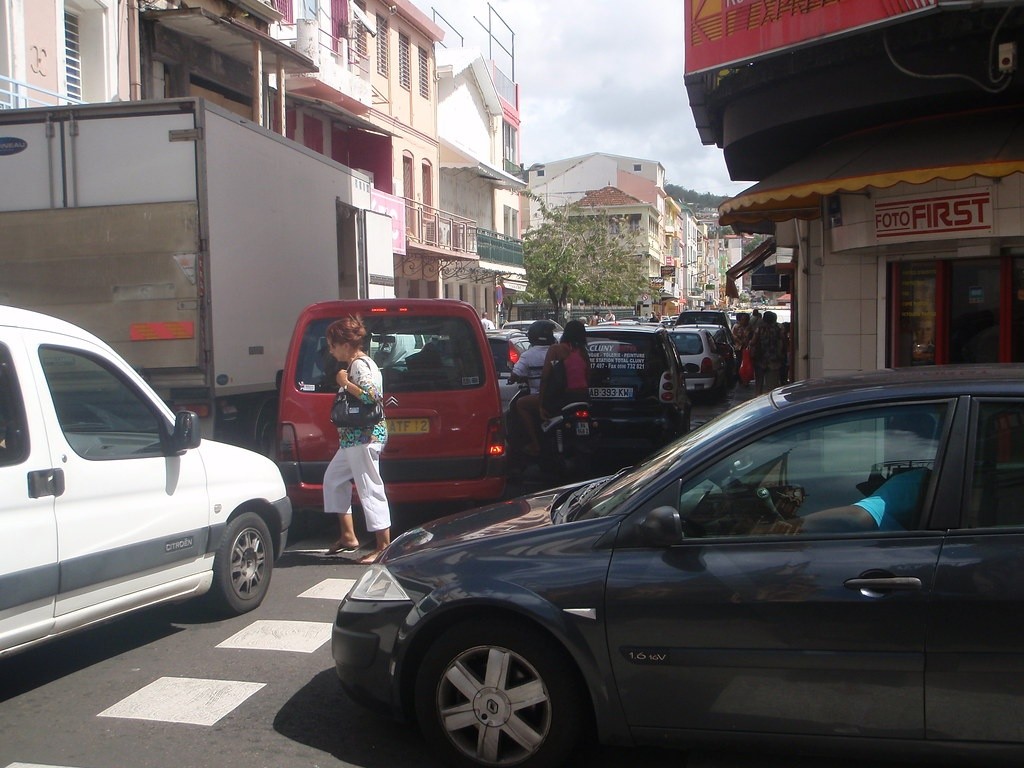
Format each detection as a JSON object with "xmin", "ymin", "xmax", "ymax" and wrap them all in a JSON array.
[{"xmin": 332, "ymin": 343, "xmax": 337, "ymax": 349}]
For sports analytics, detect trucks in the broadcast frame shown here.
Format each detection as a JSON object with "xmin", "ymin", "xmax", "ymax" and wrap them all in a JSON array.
[{"xmin": 2, "ymin": 92, "xmax": 397, "ymax": 451}]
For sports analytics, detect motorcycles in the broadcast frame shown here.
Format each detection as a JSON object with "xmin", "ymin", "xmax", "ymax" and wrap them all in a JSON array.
[{"xmin": 501, "ymin": 373, "xmax": 604, "ymax": 489}]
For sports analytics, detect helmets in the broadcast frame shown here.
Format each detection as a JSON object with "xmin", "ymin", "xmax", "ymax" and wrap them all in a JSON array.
[{"xmin": 528, "ymin": 319, "xmax": 556, "ymax": 345}]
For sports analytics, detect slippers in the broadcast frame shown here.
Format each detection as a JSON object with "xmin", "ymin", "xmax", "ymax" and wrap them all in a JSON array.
[
  {"xmin": 325, "ymin": 541, "xmax": 359, "ymax": 555},
  {"xmin": 358, "ymin": 551, "xmax": 378, "ymax": 564}
]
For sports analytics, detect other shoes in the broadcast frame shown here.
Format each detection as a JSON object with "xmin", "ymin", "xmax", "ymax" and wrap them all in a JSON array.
[{"xmin": 520, "ymin": 445, "xmax": 540, "ymax": 456}]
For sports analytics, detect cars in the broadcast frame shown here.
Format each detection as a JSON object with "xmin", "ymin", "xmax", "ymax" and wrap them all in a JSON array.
[
  {"xmin": 484, "ymin": 304, "xmax": 753, "ymax": 479},
  {"xmin": 328, "ymin": 361, "xmax": 1024, "ymax": 768}
]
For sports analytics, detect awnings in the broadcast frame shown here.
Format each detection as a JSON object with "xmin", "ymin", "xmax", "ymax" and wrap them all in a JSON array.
[
  {"xmin": 750, "ymin": 265, "xmax": 792, "ymax": 292},
  {"xmin": 724, "ymin": 236, "xmax": 777, "ymax": 298},
  {"xmin": 717, "ymin": 103, "xmax": 1024, "ymax": 226}
]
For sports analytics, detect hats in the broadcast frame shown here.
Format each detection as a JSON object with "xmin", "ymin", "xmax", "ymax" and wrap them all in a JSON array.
[{"xmin": 763, "ymin": 311, "xmax": 777, "ymax": 322}]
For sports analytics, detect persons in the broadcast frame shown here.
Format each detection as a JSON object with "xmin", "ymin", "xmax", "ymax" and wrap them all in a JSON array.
[
  {"xmin": 322, "ymin": 313, "xmax": 790, "ymax": 566},
  {"xmin": 770, "ymin": 413, "xmax": 934, "ymax": 535}
]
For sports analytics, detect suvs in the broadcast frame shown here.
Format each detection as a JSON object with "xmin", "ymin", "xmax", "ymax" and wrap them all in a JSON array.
[{"xmin": 0, "ymin": 303, "xmax": 294, "ymax": 665}]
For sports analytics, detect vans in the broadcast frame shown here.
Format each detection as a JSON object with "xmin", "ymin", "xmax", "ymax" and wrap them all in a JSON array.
[{"xmin": 274, "ymin": 297, "xmax": 511, "ymax": 544}]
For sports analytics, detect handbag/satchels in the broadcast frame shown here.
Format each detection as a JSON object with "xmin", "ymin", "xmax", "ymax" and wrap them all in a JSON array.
[
  {"xmin": 739, "ymin": 346, "xmax": 754, "ymax": 382},
  {"xmin": 549, "ymin": 344, "xmax": 566, "ymax": 391},
  {"xmin": 564, "ymin": 342, "xmax": 588, "ymax": 389},
  {"xmin": 329, "ymin": 357, "xmax": 382, "ymax": 428}
]
